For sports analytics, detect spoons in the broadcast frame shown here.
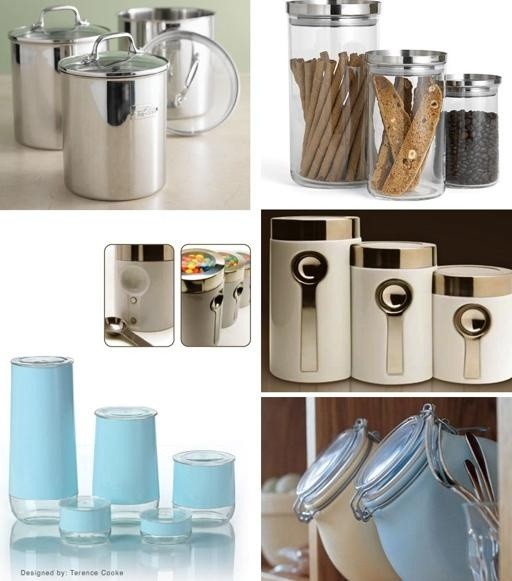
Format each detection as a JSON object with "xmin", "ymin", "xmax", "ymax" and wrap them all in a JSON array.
[{"xmin": 104, "ymin": 317, "xmax": 153, "ymax": 346}]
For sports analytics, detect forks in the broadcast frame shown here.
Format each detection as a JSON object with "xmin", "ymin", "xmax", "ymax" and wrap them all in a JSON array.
[{"xmin": 419, "ymin": 411, "xmax": 498, "ymax": 528}]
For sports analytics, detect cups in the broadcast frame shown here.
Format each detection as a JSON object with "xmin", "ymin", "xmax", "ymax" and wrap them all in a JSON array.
[
  {"xmin": 459, "ymin": 502, "xmax": 498, "ymax": 580},
  {"xmin": 57, "ymin": 495, "xmax": 113, "ymax": 546},
  {"xmin": 136, "ymin": 506, "xmax": 193, "ymax": 545}
]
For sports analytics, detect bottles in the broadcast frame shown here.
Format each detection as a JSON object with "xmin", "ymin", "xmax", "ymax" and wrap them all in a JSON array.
[
  {"xmin": 431, "ymin": 264, "xmax": 512, "ymax": 385},
  {"xmin": 179, "ymin": 249, "xmax": 249, "ymax": 347},
  {"xmin": 362, "ymin": 47, "xmax": 450, "ymax": 201},
  {"xmin": 351, "ymin": 402, "xmax": 498, "ymax": 579},
  {"xmin": 89, "ymin": 405, "xmax": 161, "ymax": 527},
  {"xmin": 7, "ymin": 354, "xmax": 81, "ymax": 525},
  {"xmin": 170, "ymin": 450, "xmax": 238, "ymax": 528},
  {"xmin": 343, "ymin": 240, "xmax": 438, "ymax": 385},
  {"xmin": 285, "ymin": 0, "xmax": 381, "ymax": 190},
  {"xmin": 267, "ymin": 213, "xmax": 362, "ymax": 385},
  {"xmin": 292, "ymin": 404, "xmax": 401, "ymax": 581},
  {"xmin": 435, "ymin": 71, "xmax": 503, "ymax": 190},
  {"xmin": 107, "ymin": 245, "xmax": 173, "ymax": 332}
]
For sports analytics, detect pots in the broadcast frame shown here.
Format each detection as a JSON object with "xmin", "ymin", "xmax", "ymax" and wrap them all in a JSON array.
[
  {"xmin": 57, "ymin": 30, "xmax": 170, "ymax": 203},
  {"xmin": 7, "ymin": 3, "xmax": 109, "ymax": 151},
  {"xmin": 120, "ymin": 7, "xmax": 240, "ymax": 135}
]
